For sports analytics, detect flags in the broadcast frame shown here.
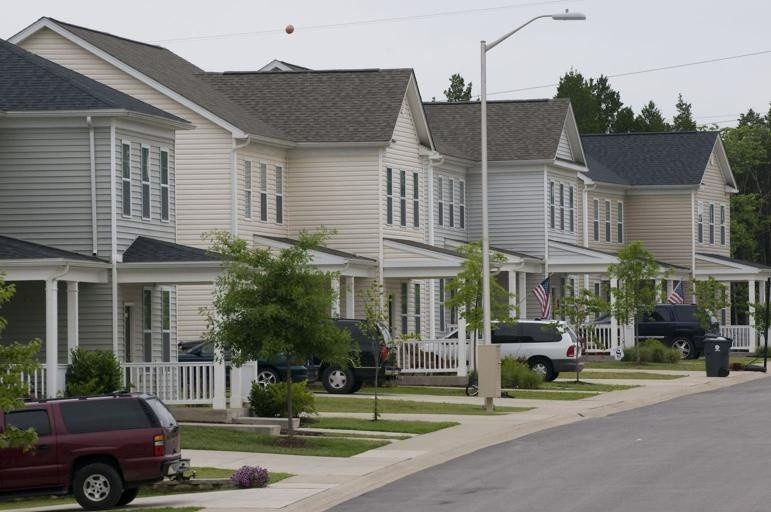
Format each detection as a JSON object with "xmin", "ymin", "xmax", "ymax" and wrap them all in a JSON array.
[
  {"xmin": 668, "ymin": 282, "xmax": 685, "ymax": 305},
  {"xmin": 531, "ymin": 276, "xmax": 552, "ymax": 321}
]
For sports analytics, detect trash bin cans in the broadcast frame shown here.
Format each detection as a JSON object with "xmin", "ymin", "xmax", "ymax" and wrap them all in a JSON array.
[{"xmin": 703, "ymin": 334, "xmax": 733, "ymax": 377}]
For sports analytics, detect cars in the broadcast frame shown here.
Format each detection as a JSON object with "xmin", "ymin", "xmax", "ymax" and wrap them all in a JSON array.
[{"xmin": 179, "ymin": 334, "xmax": 317, "ymax": 394}]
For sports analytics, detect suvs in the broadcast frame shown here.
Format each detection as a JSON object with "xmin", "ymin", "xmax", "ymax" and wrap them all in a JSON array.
[
  {"xmin": 179, "ymin": 317, "xmax": 397, "ymax": 394},
  {"xmin": 420, "ymin": 316, "xmax": 588, "ymax": 382},
  {"xmin": 0, "ymin": 390, "xmax": 180, "ymax": 511},
  {"xmin": 587, "ymin": 303, "xmax": 723, "ymax": 362}
]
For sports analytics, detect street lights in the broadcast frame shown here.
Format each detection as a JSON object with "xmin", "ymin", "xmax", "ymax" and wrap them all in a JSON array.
[{"xmin": 476, "ymin": 8, "xmax": 588, "ymax": 410}]
